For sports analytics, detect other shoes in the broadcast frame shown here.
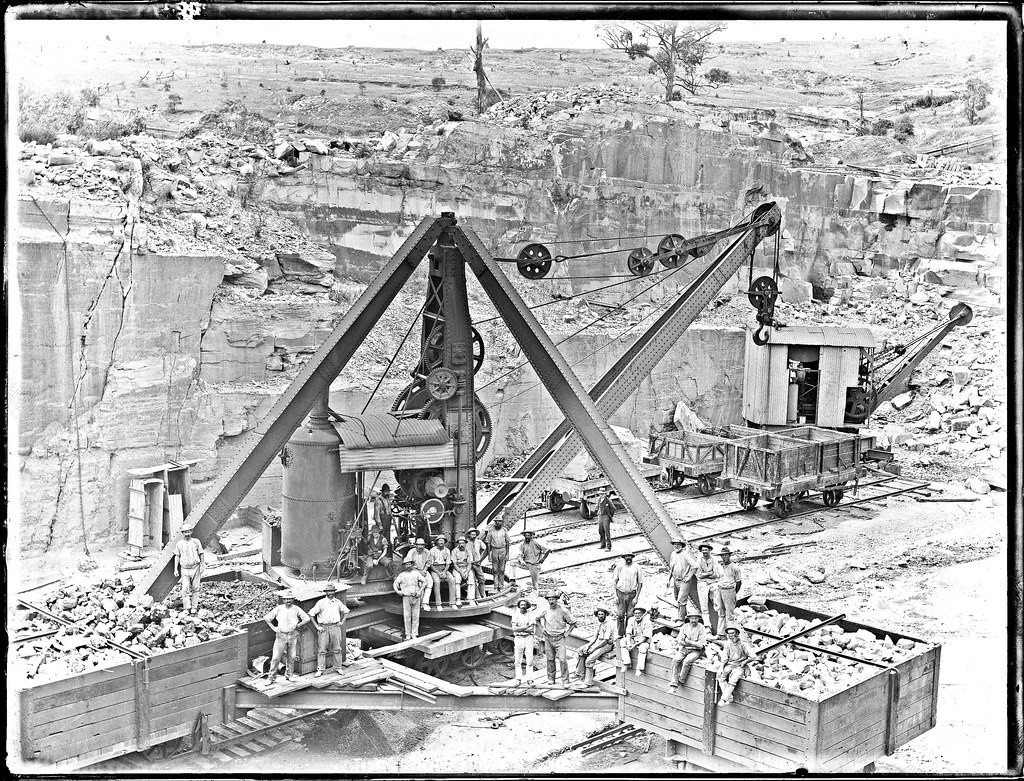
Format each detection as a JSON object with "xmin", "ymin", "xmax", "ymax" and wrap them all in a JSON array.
[
  {"xmin": 507, "ymin": 667, "xmax": 744, "ymax": 708},
  {"xmin": 605, "ymin": 548, "xmax": 610, "ymax": 552},
  {"xmin": 598, "ymin": 546, "xmax": 604, "ymax": 548},
  {"xmin": 265, "ymin": 675, "xmax": 295, "ymax": 686},
  {"xmin": 315, "ymin": 669, "xmax": 345, "ymax": 677},
  {"xmin": 358, "ymin": 574, "xmax": 540, "ymax": 641}
]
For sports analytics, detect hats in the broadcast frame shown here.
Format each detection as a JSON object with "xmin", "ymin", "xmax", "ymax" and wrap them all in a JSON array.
[
  {"xmin": 632, "ymin": 606, "xmax": 646, "ymax": 614},
  {"xmin": 180, "ymin": 524, "xmax": 193, "ymax": 531},
  {"xmin": 401, "ymin": 557, "xmax": 416, "ymax": 565},
  {"xmin": 669, "ymin": 537, "xmax": 687, "ymax": 548},
  {"xmin": 280, "ymin": 590, "xmax": 296, "ymax": 599},
  {"xmin": 725, "ymin": 624, "xmax": 741, "ymax": 635},
  {"xmin": 517, "ymin": 597, "xmax": 532, "ymax": 609},
  {"xmin": 380, "ymin": 483, "xmax": 392, "ymax": 492},
  {"xmin": 519, "ymin": 529, "xmax": 535, "ymax": 535},
  {"xmin": 414, "ymin": 515, "xmax": 506, "ymax": 549},
  {"xmin": 621, "ymin": 548, "xmax": 636, "ymax": 557},
  {"xmin": 685, "ymin": 609, "xmax": 703, "ymax": 616},
  {"xmin": 697, "ymin": 542, "xmax": 714, "ymax": 551},
  {"xmin": 323, "ymin": 583, "xmax": 336, "ymax": 592},
  {"xmin": 369, "ymin": 525, "xmax": 382, "ymax": 533},
  {"xmin": 597, "ymin": 487, "xmax": 606, "ymax": 494},
  {"xmin": 545, "ymin": 592, "xmax": 560, "ymax": 600},
  {"xmin": 717, "ymin": 546, "xmax": 734, "ymax": 555},
  {"xmin": 594, "ymin": 606, "xmax": 609, "ymax": 618}
]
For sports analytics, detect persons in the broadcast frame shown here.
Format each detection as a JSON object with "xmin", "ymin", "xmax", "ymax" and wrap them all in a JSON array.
[
  {"xmin": 665, "ymin": 535, "xmax": 741, "ymax": 638},
  {"xmin": 393, "ymin": 527, "xmax": 487, "ymax": 641},
  {"xmin": 374, "ymin": 483, "xmax": 394, "ymax": 544},
  {"xmin": 715, "ymin": 621, "xmax": 757, "ymax": 705},
  {"xmin": 620, "ymin": 604, "xmax": 653, "ymax": 677},
  {"xmin": 510, "ymin": 528, "xmax": 551, "ymax": 590},
  {"xmin": 174, "ymin": 523, "xmax": 204, "ymax": 615},
  {"xmin": 264, "ymin": 588, "xmax": 311, "ymax": 686},
  {"xmin": 648, "ymin": 424, "xmax": 661, "ymax": 454},
  {"xmin": 532, "ymin": 592, "xmax": 577, "ymax": 689},
  {"xmin": 308, "ymin": 584, "xmax": 351, "ymax": 678},
  {"xmin": 361, "ymin": 525, "xmax": 396, "ymax": 585},
  {"xmin": 484, "ymin": 514, "xmax": 510, "ymax": 590},
  {"xmin": 667, "ymin": 609, "xmax": 706, "ymax": 694},
  {"xmin": 612, "ymin": 549, "xmax": 643, "ymax": 639},
  {"xmin": 575, "ymin": 606, "xmax": 615, "ymax": 688},
  {"xmin": 511, "ymin": 597, "xmax": 536, "ymax": 688},
  {"xmin": 594, "ymin": 487, "xmax": 617, "ymax": 552},
  {"xmin": 792, "ymin": 363, "xmax": 811, "ymax": 396}
]
